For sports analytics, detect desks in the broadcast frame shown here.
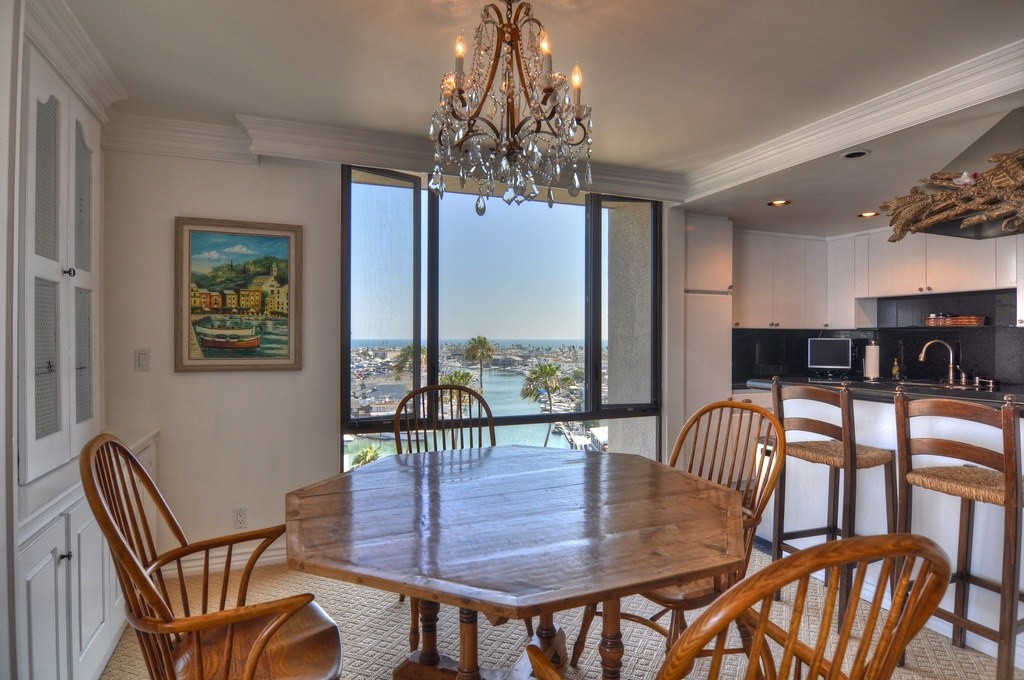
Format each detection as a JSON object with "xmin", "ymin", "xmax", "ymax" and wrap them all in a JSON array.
[{"xmin": 284, "ymin": 444, "xmax": 748, "ymax": 680}]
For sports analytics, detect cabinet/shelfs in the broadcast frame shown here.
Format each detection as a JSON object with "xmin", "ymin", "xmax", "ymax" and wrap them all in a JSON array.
[
  {"xmin": 10, "ymin": 0, "xmax": 156, "ymax": 680},
  {"xmin": 683, "ymin": 214, "xmax": 1024, "ymax": 486}
]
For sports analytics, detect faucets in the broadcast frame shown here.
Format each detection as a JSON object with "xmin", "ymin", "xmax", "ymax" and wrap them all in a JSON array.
[{"xmin": 918, "ymin": 339, "xmax": 955, "ymax": 384}]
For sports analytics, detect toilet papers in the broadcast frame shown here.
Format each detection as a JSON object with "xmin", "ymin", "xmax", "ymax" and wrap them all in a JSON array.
[{"xmin": 865, "ymin": 345, "xmax": 879, "ymax": 378}]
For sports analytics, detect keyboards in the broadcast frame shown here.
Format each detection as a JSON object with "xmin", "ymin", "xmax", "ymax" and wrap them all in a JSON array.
[{"xmin": 808, "ymin": 379, "xmax": 852, "ymax": 383}]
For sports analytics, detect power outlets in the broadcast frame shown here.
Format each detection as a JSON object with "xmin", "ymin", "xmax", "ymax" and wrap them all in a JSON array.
[{"xmin": 233, "ymin": 507, "xmax": 248, "ymax": 530}]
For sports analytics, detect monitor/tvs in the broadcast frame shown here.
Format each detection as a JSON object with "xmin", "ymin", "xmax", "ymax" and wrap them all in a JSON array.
[{"xmin": 808, "ymin": 337, "xmax": 851, "ymax": 378}]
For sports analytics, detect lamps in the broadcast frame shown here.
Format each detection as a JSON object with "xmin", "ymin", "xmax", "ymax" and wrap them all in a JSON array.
[{"xmin": 428, "ymin": 0, "xmax": 593, "ymax": 217}]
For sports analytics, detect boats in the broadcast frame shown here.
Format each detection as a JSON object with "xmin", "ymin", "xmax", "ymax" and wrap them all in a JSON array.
[
  {"xmin": 490, "ymin": 364, "xmax": 525, "ymax": 373},
  {"xmin": 539, "ymin": 394, "xmax": 575, "ymax": 435}
]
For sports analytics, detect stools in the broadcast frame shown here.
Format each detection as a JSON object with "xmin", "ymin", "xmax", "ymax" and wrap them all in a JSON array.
[
  {"xmin": 893, "ymin": 386, "xmax": 1024, "ymax": 680},
  {"xmin": 770, "ymin": 374, "xmax": 898, "ymax": 634}
]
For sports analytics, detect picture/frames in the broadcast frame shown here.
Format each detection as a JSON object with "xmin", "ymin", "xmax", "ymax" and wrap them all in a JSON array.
[{"xmin": 175, "ymin": 216, "xmax": 303, "ymax": 370}]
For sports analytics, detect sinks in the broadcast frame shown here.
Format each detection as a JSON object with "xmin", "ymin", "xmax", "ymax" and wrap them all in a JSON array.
[{"xmin": 883, "ymin": 381, "xmax": 945, "ymax": 389}]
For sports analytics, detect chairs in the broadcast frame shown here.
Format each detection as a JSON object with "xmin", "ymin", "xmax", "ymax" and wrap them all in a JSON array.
[
  {"xmin": 571, "ymin": 400, "xmax": 786, "ymax": 679},
  {"xmin": 526, "ymin": 532, "xmax": 951, "ymax": 680},
  {"xmin": 392, "ymin": 385, "xmax": 496, "ymax": 602},
  {"xmin": 79, "ymin": 434, "xmax": 342, "ymax": 680}
]
